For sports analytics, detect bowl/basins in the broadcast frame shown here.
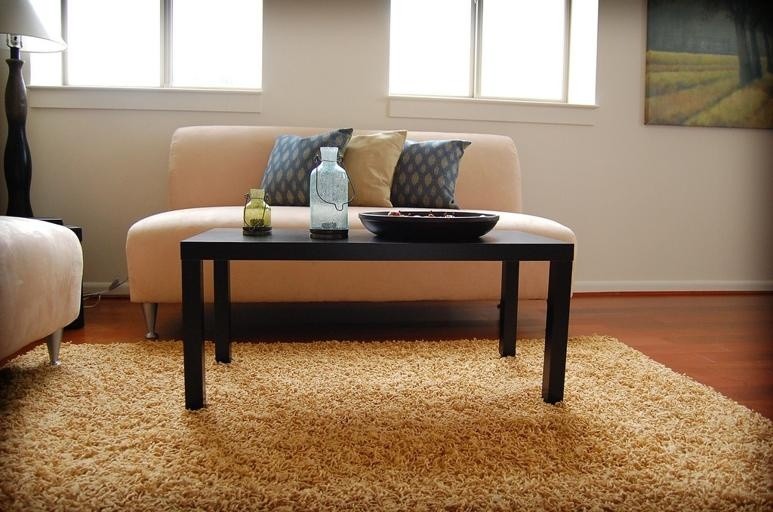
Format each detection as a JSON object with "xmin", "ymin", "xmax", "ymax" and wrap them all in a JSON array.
[{"xmin": 357, "ymin": 208, "xmax": 500, "ymax": 242}]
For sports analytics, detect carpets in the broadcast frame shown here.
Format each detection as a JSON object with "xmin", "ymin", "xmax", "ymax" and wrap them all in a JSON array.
[{"xmin": 2, "ymin": 332, "xmax": 772, "ymax": 512}]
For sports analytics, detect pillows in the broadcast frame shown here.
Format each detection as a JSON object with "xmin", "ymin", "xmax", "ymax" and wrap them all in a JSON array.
[{"xmin": 257, "ymin": 127, "xmax": 469, "ymax": 208}]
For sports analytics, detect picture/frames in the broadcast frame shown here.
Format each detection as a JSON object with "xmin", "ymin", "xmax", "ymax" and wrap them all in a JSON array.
[{"xmin": 645, "ymin": 0, "xmax": 773, "ymax": 130}]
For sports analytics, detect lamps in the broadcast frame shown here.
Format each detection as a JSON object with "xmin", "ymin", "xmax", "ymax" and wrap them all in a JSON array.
[{"xmin": 1, "ymin": 1, "xmax": 68, "ymax": 217}]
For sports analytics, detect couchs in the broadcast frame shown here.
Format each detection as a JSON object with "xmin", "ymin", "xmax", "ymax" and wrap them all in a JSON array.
[{"xmin": 125, "ymin": 127, "xmax": 575, "ymax": 338}]
